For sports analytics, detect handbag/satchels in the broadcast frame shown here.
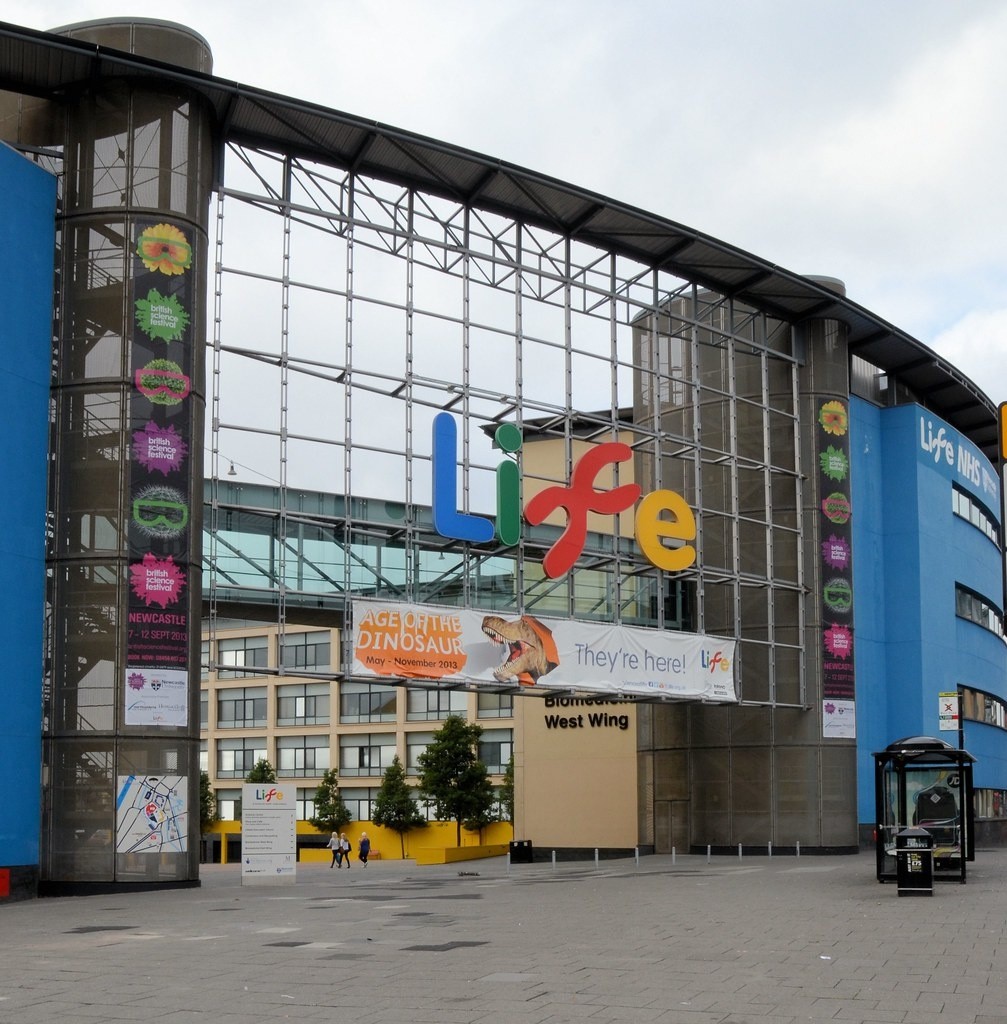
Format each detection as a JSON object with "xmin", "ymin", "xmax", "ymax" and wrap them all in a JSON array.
[
  {"xmin": 347, "ymin": 843, "xmax": 352, "ymax": 852},
  {"xmin": 338, "ymin": 847, "xmax": 344, "ymax": 854}
]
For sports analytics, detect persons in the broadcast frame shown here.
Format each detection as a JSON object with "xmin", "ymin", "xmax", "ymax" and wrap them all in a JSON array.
[
  {"xmin": 359, "ymin": 832, "xmax": 370, "ymax": 868},
  {"xmin": 327, "ymin": 832, "xmax": 342, "ymax": 868},
  {"xmin": 338, "ymin": 833, "xmax": 350, "ymax": 869}
]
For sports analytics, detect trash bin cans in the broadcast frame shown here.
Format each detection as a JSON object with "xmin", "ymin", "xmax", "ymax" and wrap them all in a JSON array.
[
  {"xmin": 509, "ymin": 840, "xmax": 533, "ymax": 863},
  {"xmin": 895, "ymin": 826, "xmax": 934, "ymax": 898}
]
